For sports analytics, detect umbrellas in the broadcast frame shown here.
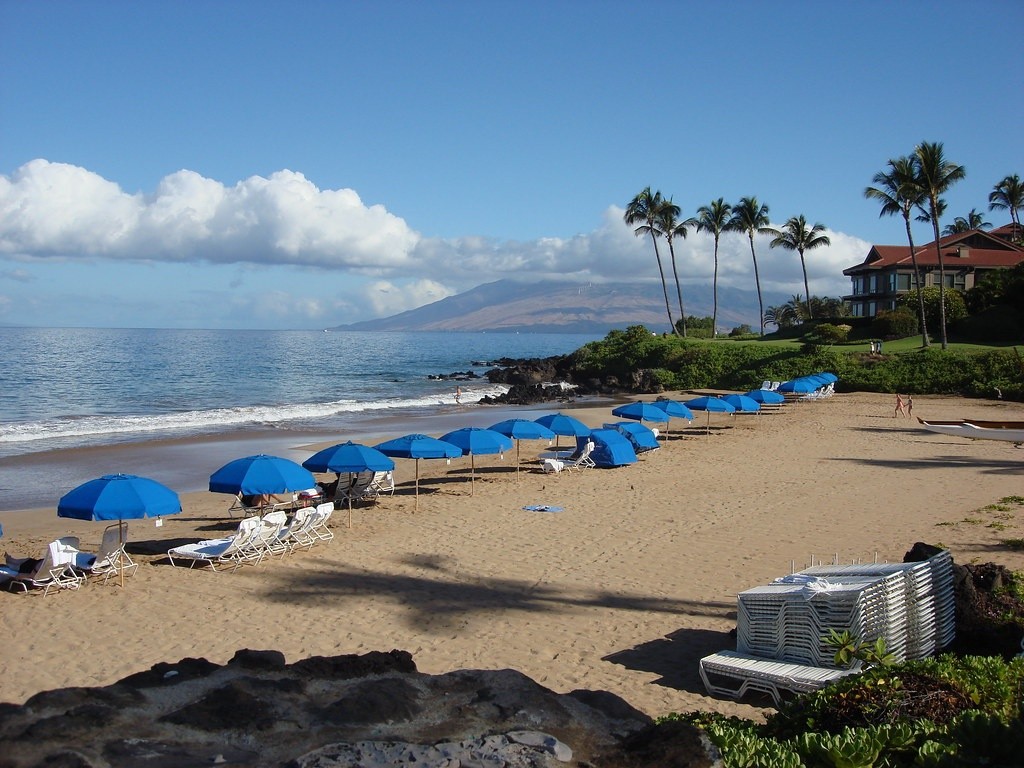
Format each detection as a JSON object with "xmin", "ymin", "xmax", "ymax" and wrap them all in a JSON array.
[
  {"xmin": 612, "ymin": 401, "xmax": 670, "ymax": 424},
  {"xmin": 486, "ymin": 418, "xmax": 556, "ymax": 482},
  {"xmin": 683, "ymin": 395, "xmax": 735, "ymax": 437},
  {"xmin": 721, "ymin": 393, "xmax": 761, "ymax": 419},
  {"xmin": 534, "ymin": 412, "xmax": 592, "ymax": 451},
  {"xmin": 777, "ymin": 372, "xmax": 838, "ymax": 401},
  {"xmin": 57, "ymin": 473, "xmax": 182, "ymax": 586},
  {"xmin": 209, "ymin": 453, "xmax": 316, "ymax": 515},
  {"xmin": 372, "ymin": 433, "xmax": 462, "ymax": 511},
  {"xmin": 745, "ymin": 390, "xmax": 784, "ymax": 404},
  {"xmin": 302, "ymin": 440, "xmax": 395, "ymax": 528},
  {"xmin": 649, "ymin": 399, "xmax": 694, "ymax": 446},
  {"xmin": 438, "ymin": 426, "xmax": 514, "ymax": 494}
]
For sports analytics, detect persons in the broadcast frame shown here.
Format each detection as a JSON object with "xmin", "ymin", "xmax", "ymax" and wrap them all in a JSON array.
[
  {"xmin": 238, "ymin": 491, "xmax": 285, "ymax": 507},
  {"xmin": 454, "ymin": 386, "xmax": 461, "ymax": 405},
  {"xmin": 652, "ymin": 331, "xmax": 656, "ymax": 336},
  {"xmin": 893, "ymin": 394, "xmax": 906, "ymax": 418},
  {"xmin": 317, "ymin": 472, "xmax": 339, "ymax": 495},
  {"xmin": 663, "ymin": 330, "xmax": 668, "ymax": 338},
  {"xmin": 351, "ymin": 472, "xmax": 358, "ymax": 488},
  {"xmin": 870, "ymin": 342, "xmax": 875, "ymax": 355},
  {"xmin": 905, "ymin": 395, "xmax": 913, "ymax": 418},
  {"xmin": 876, "ymin": 341, "xmax": 881, "ymax": 354}
]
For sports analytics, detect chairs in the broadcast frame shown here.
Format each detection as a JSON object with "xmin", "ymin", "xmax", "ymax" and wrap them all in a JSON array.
[
  {"xmin": 760, "ymin": 381, "xmax": 835, "ymax": 402},
  {"xmin": 168, "ymin": 471, "xmax": 394, "ymax": 573},
  {"xmin": 698, "ymin": 549, "xmax": 956, "ymax": 708},
  {"xmin": 538, "ymin": 442, "xmax": 596, "ymax": 475},
  {"xmin": 0, "ymin": 522, "xmax": 138, "ymax": 598}
]
